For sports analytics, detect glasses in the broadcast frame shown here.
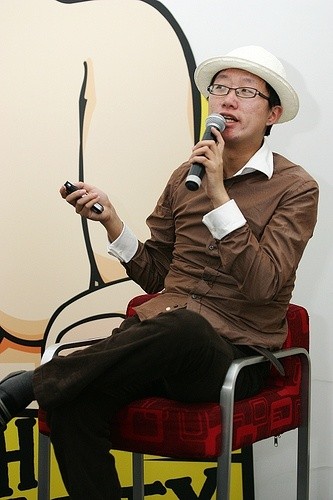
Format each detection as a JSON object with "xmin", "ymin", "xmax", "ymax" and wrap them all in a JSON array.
[{"xmin": 207, "ymin": 84, "xmax": 274, "ymax": 104}]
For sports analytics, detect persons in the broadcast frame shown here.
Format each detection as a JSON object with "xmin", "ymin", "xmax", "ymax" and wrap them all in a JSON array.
[{"xmin": 1, "ymin": 45, "xmax": 319, "ymax": 499}]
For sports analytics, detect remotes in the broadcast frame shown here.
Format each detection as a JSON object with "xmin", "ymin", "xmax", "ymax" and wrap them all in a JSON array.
[{"xmin": 64, "ymin": 181, "xmax": 104, "ymax": 214}]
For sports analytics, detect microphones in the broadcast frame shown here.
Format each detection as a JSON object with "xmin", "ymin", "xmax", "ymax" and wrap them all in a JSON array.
[{"xmin": 185, "ymin": 113, "xmax": 226, "ymax": 192}]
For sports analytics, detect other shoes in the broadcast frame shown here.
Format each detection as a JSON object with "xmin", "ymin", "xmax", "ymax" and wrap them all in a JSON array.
[{"xmin": 0, "ymin": 370, "xmax": 33, "ymax": 431}]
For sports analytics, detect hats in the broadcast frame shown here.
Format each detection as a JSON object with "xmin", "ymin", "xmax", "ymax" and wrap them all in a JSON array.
[{"xmin": 194, "ymin": 45, "xmax": 300, "ymax": 125}]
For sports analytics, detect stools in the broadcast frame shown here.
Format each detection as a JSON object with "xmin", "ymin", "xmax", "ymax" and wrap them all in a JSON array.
[{"xmin": 38, "ymin": 292, "xmax": 310, "ymax": 500}]
